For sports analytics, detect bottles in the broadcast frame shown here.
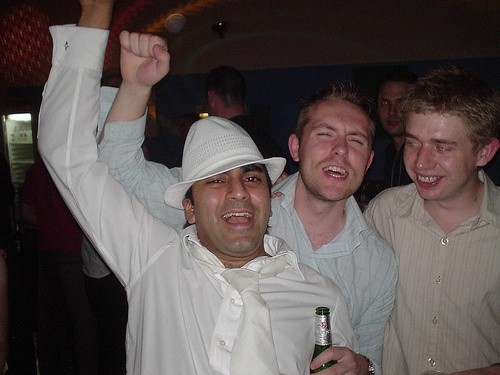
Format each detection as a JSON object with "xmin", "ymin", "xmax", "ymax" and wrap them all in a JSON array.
[{"xmin": 310, "ymin": 307, "xmax": 338, "ymax": 374}]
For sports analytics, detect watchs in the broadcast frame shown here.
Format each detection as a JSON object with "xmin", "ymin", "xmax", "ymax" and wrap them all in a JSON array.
[{"xmin": 362, "ymin": 355, "xmax": 376, "ymax": 375}]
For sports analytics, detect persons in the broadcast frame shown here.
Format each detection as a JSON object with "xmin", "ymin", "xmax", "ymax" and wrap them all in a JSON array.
[
  {"xmin": 360, "ymin": 74, "xmax": 413, "ymax": 205},
  {"xmin": 98, "ymin": 31, "xmax": 399, "ymax": 375},
  {"xmin": 1, "ymin": 32, "xmax": 384, "ymax": 375},
  {"xmin": 38, "ymin": 0, "xmax": 358, "ymax": 375},
  {"xmin": 271, "ymin": 62, "xmax": 500, "ymax": 375}
]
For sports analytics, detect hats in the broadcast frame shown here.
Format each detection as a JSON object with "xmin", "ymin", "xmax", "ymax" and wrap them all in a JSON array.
[{"xmin": 164, "ymin": 116, "xmax": 286, "ymax": 210}]
